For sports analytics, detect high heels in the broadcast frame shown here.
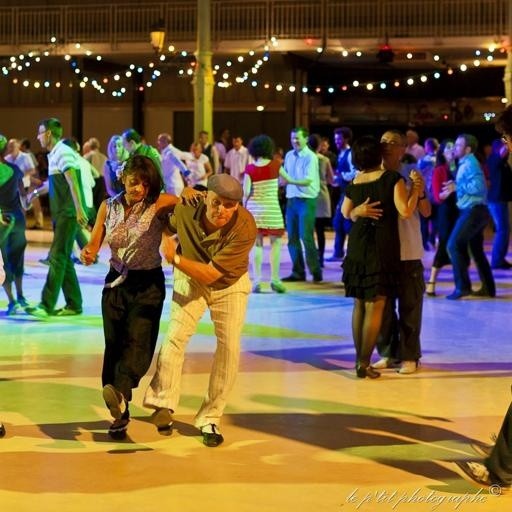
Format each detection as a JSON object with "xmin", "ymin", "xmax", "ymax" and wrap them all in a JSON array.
[{"xmin": 357, "ymin": 366, "xmax": 380, "ymax": 378}]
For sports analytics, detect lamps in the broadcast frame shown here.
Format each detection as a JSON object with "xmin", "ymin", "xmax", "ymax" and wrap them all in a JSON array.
[{"xmin": 150, "ymin": 31, "xmax": 165, "ymax": 59}]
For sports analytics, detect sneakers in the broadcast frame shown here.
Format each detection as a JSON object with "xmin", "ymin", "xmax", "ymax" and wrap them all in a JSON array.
[
  {"xmin": 400, "ymin": 360, "xmax": 421, "ymax": 374},
  {"xmin": 371, "ymin": 357, "xmax": 401, "ymax": 369},
  {"xmin": 453, "ymin": 461, "xmax": 512, "ymax": 495},
  {"xmin": 7, "ymin": 298, "xmax": 82, "ymax": 318}
]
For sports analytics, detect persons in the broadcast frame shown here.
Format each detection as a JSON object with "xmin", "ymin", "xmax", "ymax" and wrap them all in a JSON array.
[
  {"xmin": 454, "ymin": 383, "xmax": 512, "ymax": 488},
  {"xmin": 338, "ymin": 137, "xmax": 423, "ymax": 376},
  {"xmin": 143, "ymin": 175, "xmax": 258, "ymax": 447},
  {"xmin": 350, "ymin": 126, "xmax": 432, "ymax": 373},
  {"xmin": 1, "ymin": 117, "xmax": 512, "ymax": 317},
  {"xmin": 78, "ymin": 156, "xmax": 205, "ymax": 441}
]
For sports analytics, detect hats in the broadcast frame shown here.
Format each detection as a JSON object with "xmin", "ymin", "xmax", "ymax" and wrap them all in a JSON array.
[{"xmin": 207, "ymin": 174, "xmax": 243, "ymax": 201}]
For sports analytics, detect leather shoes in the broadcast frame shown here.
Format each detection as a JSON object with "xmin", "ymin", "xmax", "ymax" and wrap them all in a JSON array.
[
  {"xmin": 253, "ymin": 271, "xmax": 495, "ymax": 299},
  {"xmin": 103, "ymin": 384, "xmax": 223, "ymax": 446}
]
